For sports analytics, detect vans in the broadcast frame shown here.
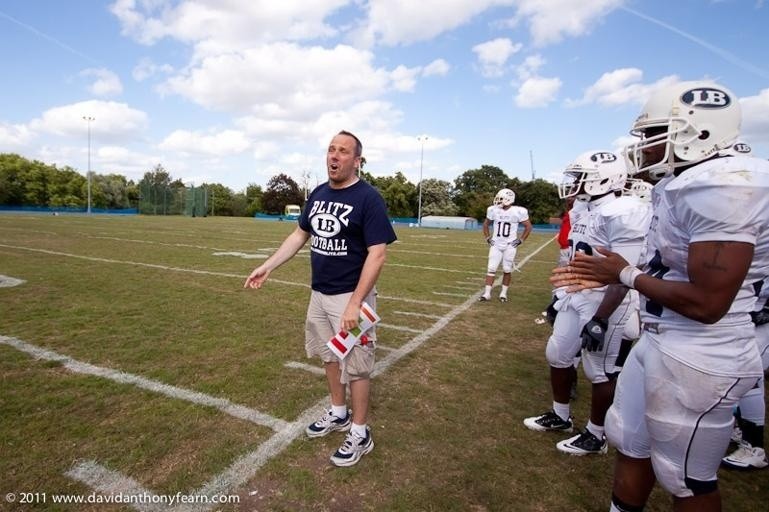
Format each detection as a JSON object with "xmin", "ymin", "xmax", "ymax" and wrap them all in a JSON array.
[{"xmin": 285, "ymin": 204, "xmax": 301, "ymax": 216}]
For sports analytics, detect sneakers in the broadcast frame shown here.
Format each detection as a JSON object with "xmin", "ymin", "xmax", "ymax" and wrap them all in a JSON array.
[
  {"xmin": 556, "ymin": 427, "xmax": 608, "ymax": 456},
  {"xmin": 720, "ymin": 425, "xmax": 769, "ymax": 470},
  {"xmin": 499, "ymin": 295, "xmax": 508, "ymax": 303},
  {"xmin": 478, "ymin": 295, "xmax": 492, "ymax": 301},
  {"xmin": 523, "ymin": 410, "xmax": 573, "ymax": 433},
  {"xmin": 329, "ymin": 426, "xmax": 374, "ymax": 467},
  {"xmin": 306, "ymin": 409, "xmax": 352, "ymax": 437}
]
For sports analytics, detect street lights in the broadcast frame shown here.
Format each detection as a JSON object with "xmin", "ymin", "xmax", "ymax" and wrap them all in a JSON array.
[
  {"xmin": 80, "ymin": 114, "xmax": 95, "ymax": 213},
  {"xmin": 415, "ymin": 136, "xmax": 430, "ymax": 226}
]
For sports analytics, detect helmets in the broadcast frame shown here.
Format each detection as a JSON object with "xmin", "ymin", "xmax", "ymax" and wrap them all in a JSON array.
[
  {"xmin": 493, "ymin": 189, "xmax": 515, "ymax": 208},
  {"xmin": 558, "ymin": 80, "xmax": 742, "ymax": 200}
]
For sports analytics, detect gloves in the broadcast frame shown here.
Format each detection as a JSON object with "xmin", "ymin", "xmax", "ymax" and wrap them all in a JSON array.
[
  {"xmin": 486, "ymin": 235, "xmax": 495, "ymax": 246},
  {"xmin": 546, "ymin": 294, "xmax": 558, "ymax": 326},
  {"xmin": 579, "ymin": 316, "xmax": 607, "ymax": 352},
  {"xmin": 511, "ymin": 239, "xmax": 521, "ymax": 248}
]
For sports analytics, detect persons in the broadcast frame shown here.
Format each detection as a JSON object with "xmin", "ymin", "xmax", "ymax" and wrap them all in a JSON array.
[
  {"xmin": 477, "ymin": 188, "xmax": 534, "ymax": 304},
  {"xmin": 522, "ymin": 79, "xmax": 768, "ymax": 510},
  {"xmin": 243, "ymin": 129, "xmax": 396, "ymax": 468}
]
